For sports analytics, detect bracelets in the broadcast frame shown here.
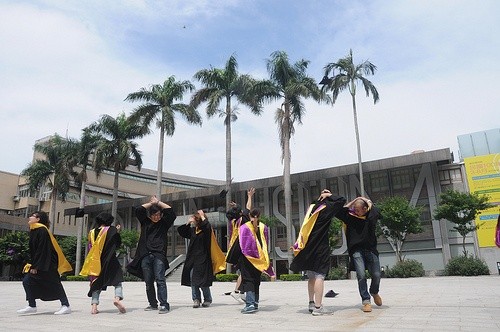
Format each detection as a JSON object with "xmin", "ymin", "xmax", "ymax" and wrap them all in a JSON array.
[
  {"xmin": 366, "ymin": 200, "xmax": 372, "ymax": 203},
  {"xmin": 157, "ymin": 200, "xmax": 161, "ymax": 205}
]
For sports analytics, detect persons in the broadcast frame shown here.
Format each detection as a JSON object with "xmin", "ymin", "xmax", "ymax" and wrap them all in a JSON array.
[
  {"xmin": 238, "ymin": 208, "xmax": 275, "ymax": 314},
  {"xmin": 225, "ymin": 187, "xmax": 256, "ymax": 303},
  {"xmin": 17, "ymin": 211, "xmax": 72, "ymax": 314},
  {"xmin": 335, "ymin": 196, "xmax": 383, "ymax": 312},
  {"xmin": 495, "ymin": 214, "xmax": 500, "ymax": 249},
  {"xmin": 177, "ymin": 209, "xmax": 226, "ymax": 308},
  {"xmin": 288, "ymin": 189, "xmax": 347, "ymax": 315},
  {"xmin": 78, "ymin": 212, "xmax": 127, "ymax": 314},
  {"xmin": 125, "ymin": 195, "xmax": 177, "ymax": 313}
]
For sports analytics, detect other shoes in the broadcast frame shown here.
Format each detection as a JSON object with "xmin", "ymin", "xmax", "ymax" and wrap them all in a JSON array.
[
  {"xmin": 17, "ymin": 306, "xmax": 37, "ymax": 314},
  {"xmin": 202, "ymin": 302, "xmax": 211, "ymax": 308},
  {"xmin": 193, "ymin": 298, "xmax": 200, "ymax": 308},
  {"xmin": 240, "ymin": 303, "xmax": 258, "ymax": 313},
  {"xmin": 309, "ymin": 302, "xmax": 315, "ymax": 311},
  {"xmin": 312, "ymin": 306, "xmax": 331, "ymax": 315},
  {"xmin": 364, "ymin": 302, "xmax": 371, "ymax": 312},
  {"xmin": 145, "ymin": 305, "xmax": 157, "ymax": 311},
  {"xmin": 54, "ymin": 305, "xmax": 70, "ymax": 315},
  {"xmin": 158, "ymin": 306, "xmax": 167, "ymax": 314},
  {"xmin": 373, "ymin": 294, "xmax": 382, "ymax": 306}
]
